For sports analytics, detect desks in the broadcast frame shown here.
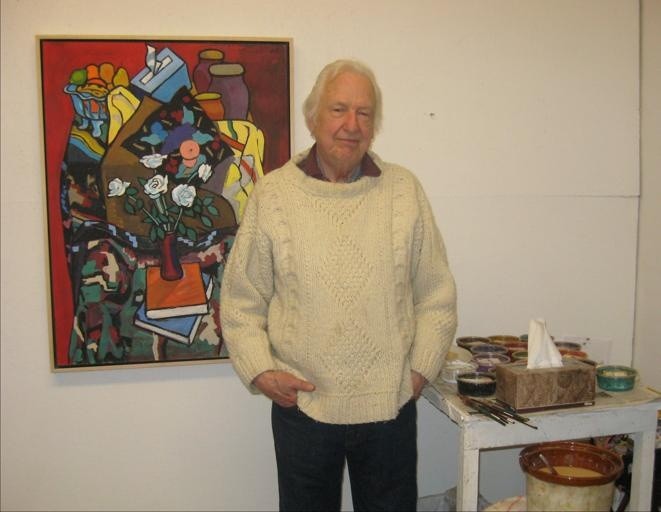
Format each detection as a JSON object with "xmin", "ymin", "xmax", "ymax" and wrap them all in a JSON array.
[{"xmin": 415, "ymin": 377, "xmax": 661, "ymax": 512}]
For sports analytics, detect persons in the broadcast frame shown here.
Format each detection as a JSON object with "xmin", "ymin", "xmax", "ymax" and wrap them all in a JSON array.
[{"xmin": 222, "ymin": 55, "xmax": 460, "ymax": 511}]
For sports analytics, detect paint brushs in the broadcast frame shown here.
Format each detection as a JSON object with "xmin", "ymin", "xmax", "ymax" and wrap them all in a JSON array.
[
  {"xmin": 457, "ymin": 394, "xmax": 539, "ymax": 429},
  {"xmin": 516, "ymin": 401, "xmax": 596, "ymax": 413}
]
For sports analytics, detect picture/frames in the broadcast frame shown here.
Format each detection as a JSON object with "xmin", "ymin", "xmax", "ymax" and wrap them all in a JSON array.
[{"xmin": 34, "ymin": 33, "xmax": 297, "ymax": 376}]
